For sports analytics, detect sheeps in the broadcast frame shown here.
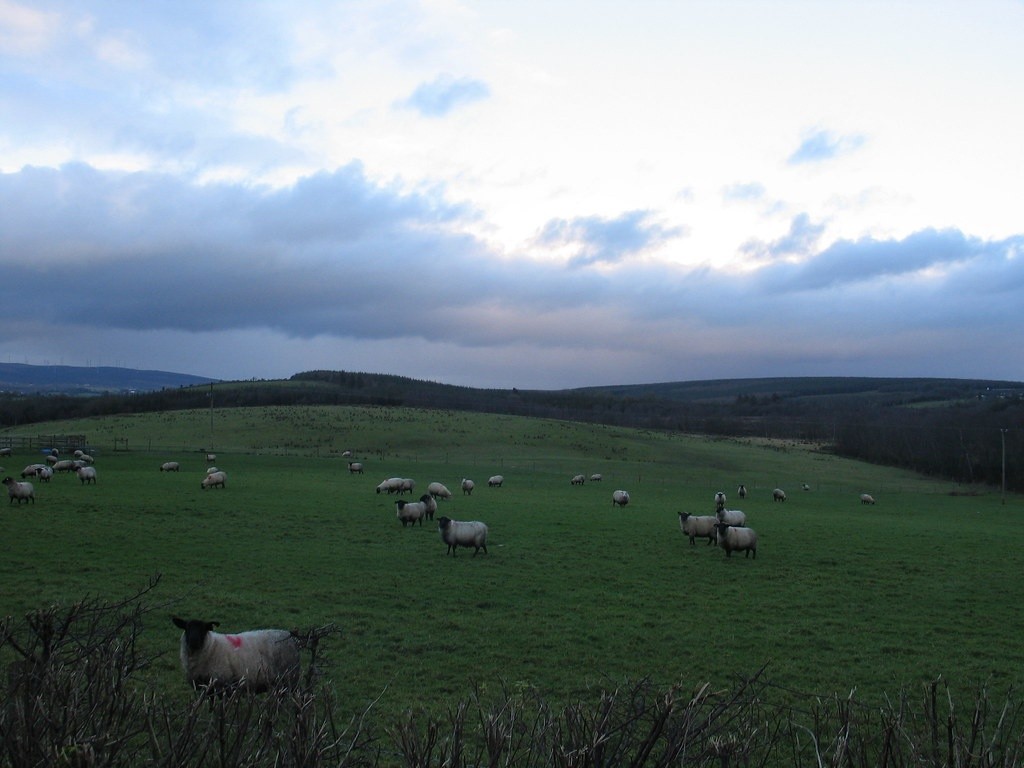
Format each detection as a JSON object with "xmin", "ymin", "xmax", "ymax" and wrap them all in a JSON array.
[
  {"xmin": 802, "ymin": 484, "xmax": 810, "ymax": 490},
  {"xmin": 0, "ymin": 447, "xmax": 97, "ymax": 485},
  {"xmin": 612, "ymin": 490, "xmax": 629, "ymax": 507},
  {"xmin": 462, "ymin": 478, "xmax": 475, "ymax": 496},
  {"xmin": 738, "ymin": 485, "xmax": 746, "ymax": 498},
  {"xmin": 715, "ymin": 492, "xmax": 727, "ymax": 507},
  {"xmin": 342, "ymin": 451, "xmax": 364, "ymax": 474},
  {"xmin": 394, "ymin": 494, "xmax": 438, "ymax": 527},
  {"xmin": 160, "ymin": 454, "xmax": 227, "ymax": 489},
  {"xmin": 714, "ymin": 522, "xmax": 757, "ymax": 560},
  {"xmin": 773, "ymin": 488, "xmax": 787, "ymax": 503},
  {"xmin": 436, "ymin": 516, "xmax": 488, "ymax": 559},
  {"xmin": 2, "ymin": 477, "xmax": 36, "ymax": 504},
  {"xmin": 488, "ymin": 475, "xmax": 505, "ymax": 487},
  {"xmin": 571, "ymin": 473, "xmax": 602, "ymax": 486},
  {"xmin": 860, "ymin": 493, "xmax": 875, "ymax": 505},
  {"xmin": 716, "ymin": 506, "xmax": 746, "ymax": 528},
  {"xmin": 171, "ymin": 615, "xmax": 300, "ymax": 714},
  {"xmin": 428, "ymin": 482, "xmax": 452, "ymax": 500},
  {"xmin": 677, "ymin": 511, "xmax": 720, "ymax": 546},
  {"xmin": 376, "ymin": 478, "xmax": 416, "ymax": 495}
]
